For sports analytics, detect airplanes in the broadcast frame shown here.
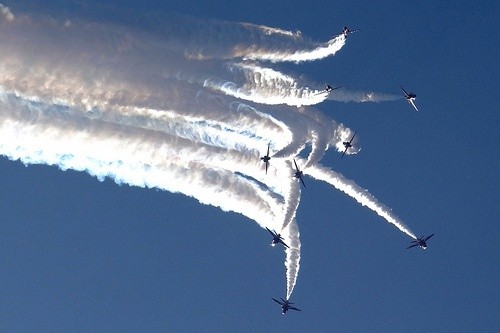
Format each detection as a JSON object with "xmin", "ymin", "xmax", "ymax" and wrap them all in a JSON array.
[
  {"xmin": 406, "ymin": 234, "xmax": 434, "ymax": 249},
  {"xmin": 271, "ymin": 297, "xmax": 300, "ymax": 314},
  {"xmin": 293, "ymin": 159, "xmax": 306, "ymax": 188},
  {"xmin": 266, "ymin": 227, "xmax": 290, "ymax": 248},
  {"xmin": 331, "ymin": 27, "xmax": 358, "ymax": 39},
  {"xmin": 261, "ymin": 143, "xmax": 270, "ymax": 174},
  {"xmin": 400, "ymin": 86, "xmax": 418, "ymax": 112},
  {"xmin": 314, "ymin": 84, "xmax": 344, "ymax": 96},
  {"xmin": 341, "ymin": 132, "xmax": 356, "ymax": 160}
]
